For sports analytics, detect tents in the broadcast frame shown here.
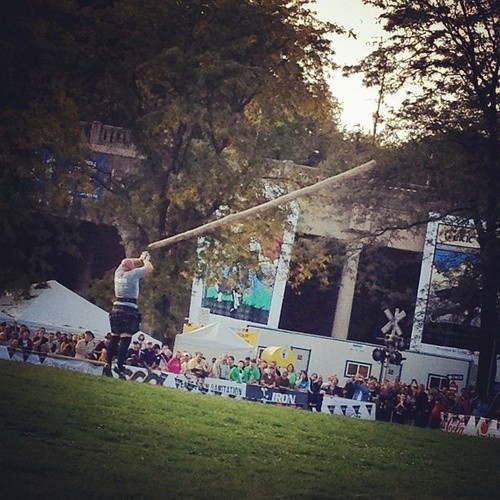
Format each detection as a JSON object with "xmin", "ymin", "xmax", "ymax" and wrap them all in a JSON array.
[{"xmin": 0, "ymin": 281, "xmax": 164, "ymax": 353}]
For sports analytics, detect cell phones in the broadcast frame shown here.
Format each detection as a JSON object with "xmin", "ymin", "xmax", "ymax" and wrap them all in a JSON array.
[{"xmin": 412, "ymin": 379, "xmax": 416, "ymax": 380}]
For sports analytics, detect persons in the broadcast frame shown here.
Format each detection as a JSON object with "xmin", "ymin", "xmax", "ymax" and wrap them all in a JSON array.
[
  {"xmin": 103, "ymin": 250, "xmax": 155, "ymax": 381},
  {"xmin": 0, "ymin": 319, "xmax": 500, "ymax": 429}
]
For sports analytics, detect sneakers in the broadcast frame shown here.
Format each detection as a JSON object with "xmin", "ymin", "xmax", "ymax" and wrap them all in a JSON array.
[
  {"xmin": 101, "ymin": 363, "xmax": 114, "ymax": 379},
  {"xmin": 113, "ymin": 364, "xmax": 126, "ymax": 379}
]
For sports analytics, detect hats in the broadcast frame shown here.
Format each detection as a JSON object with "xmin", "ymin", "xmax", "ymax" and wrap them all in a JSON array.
[
  {"xmin": 84, "ymin": 331, "xmax": 93, "ymax": 334},
  {"xmin": 104, "ymin": 333, "xmax": 113, "ymax": 339}
]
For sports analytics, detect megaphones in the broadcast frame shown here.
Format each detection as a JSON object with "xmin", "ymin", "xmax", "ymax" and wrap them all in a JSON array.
[{"xmin": 370, "ymin": 348, "xmax": 383, "ymax": 361}]
